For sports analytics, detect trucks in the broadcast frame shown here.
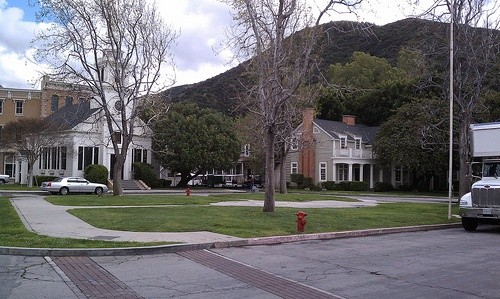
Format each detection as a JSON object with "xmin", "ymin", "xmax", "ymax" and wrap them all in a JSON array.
[{"xmin": 458, "ymin": 121, "xmax": 500, "ymax": 232}]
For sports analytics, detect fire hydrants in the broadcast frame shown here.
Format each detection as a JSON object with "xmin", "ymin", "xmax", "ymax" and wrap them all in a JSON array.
[
  {"xmin": 294, "ymin": 211, "xmax": 309, "ymax": 233},
  {"xmin": 186, "ymin": 188, "xmax": 192, "ymax": 196}
]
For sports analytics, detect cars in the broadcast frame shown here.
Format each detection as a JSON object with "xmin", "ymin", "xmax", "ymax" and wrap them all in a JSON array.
[
  {"xmin": 40, "ymin": 176, "xmax": 109, "ymax": 196},
  {"xmin": 0, "ymin": 174, "xmax": 10, "ymax": 185}
]
